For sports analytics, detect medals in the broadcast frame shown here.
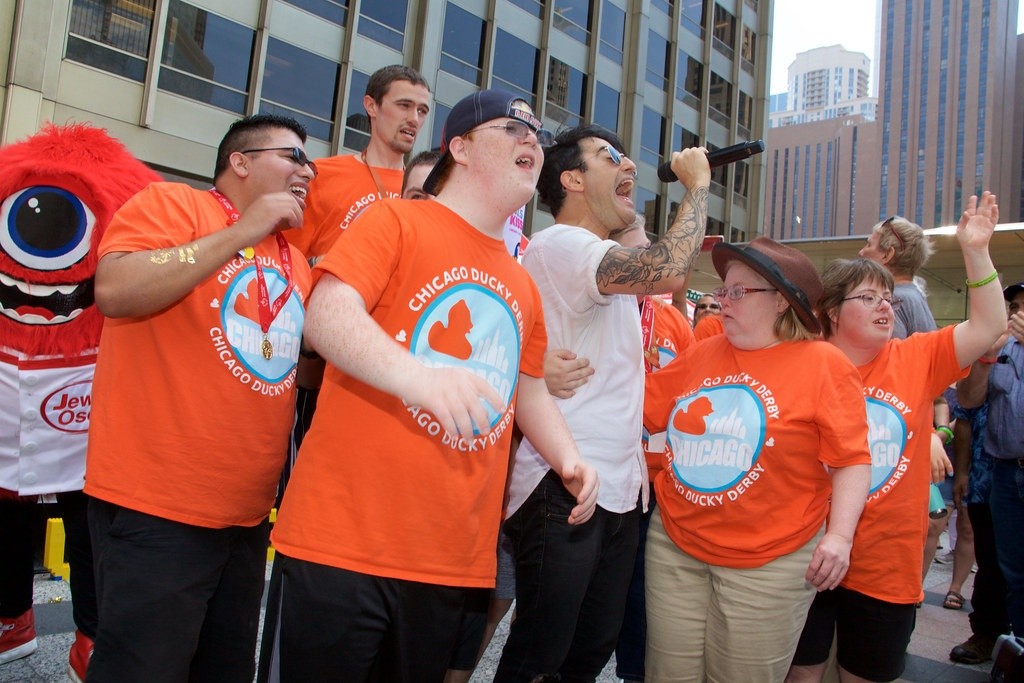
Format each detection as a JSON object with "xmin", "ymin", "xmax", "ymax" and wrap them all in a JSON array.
[{"xmin": 262, "ymin": 340, "xmax": 273, "ymax": 360}]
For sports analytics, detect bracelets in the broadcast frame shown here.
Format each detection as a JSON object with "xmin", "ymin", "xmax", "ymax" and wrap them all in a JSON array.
[
  {"xmin": 965, "ymin": 269, "xmax": 998, "ymax": 288},
  {"xmin": 978, "ymin": 357, "xmax": 999, "ymax": 364},
  {"xmin": 937, "ymin": 425, "xmax": 954, "ymax": 444}
]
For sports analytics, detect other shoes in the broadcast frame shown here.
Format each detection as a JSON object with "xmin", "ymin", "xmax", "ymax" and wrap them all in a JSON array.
[{"xmin": 937, "ymin": 546, "xmax": 943, "ymax": 549}]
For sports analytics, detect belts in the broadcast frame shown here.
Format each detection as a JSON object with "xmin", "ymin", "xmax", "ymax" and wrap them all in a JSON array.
[{"xmin": 995, "ymin": 457, "xmax": 1024, "ymax": 468}]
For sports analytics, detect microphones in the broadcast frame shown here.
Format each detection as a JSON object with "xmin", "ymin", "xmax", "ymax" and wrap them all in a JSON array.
[{"xmin": 657, "ymin": 139, "xmax": 765, "ymax": 183}]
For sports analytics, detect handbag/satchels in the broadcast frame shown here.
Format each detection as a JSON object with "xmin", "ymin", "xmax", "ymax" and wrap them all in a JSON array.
[{"xmin": 991, "ymin": 635, "xmax": 1024, "ymax": 683}]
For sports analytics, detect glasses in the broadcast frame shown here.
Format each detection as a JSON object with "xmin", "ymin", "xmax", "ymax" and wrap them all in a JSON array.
[
  {"xmin": 842, "ymin": 293, "xmax": 904, "ymax": 310},
  {"xmin": 696, "ymin": 303, "xmax": 721, "ymax": 311},
  {"xmin": 240, "ymin": 147, "xmax": 318, "ymax": 178},
  {"xmin": 882, "ymin": 216, "xmax": 905, "ymax": 256},
  {"xmin": 713, "ymin": 283, "xmax": 779, "ymax": 302},
  {"xmin": 461, "ymin": 120, "xmax": 557, "ymax": 147},
  {"xmin": 1009, "ymin": 302, "xmax": 1024, "ymax": 312},
  {"xmin": 569, "ymin": 146, "xmax": 639, "ymax": 177},
  {"xmin": 997, "ymin": 353, "xmax": 1021, "ymax": 380}
]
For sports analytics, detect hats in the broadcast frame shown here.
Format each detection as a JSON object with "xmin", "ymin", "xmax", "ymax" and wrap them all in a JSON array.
[
  {"xmin": 712, "ymin": 236, "xmax": 823, "ymax": 334},
  {"xmin": 1003, "ymin": 282, "xmax": 1024, "ymax": 302},
  {"xmin": 422, "ymin": 90, "xmax": 544, "ymax": 196}
]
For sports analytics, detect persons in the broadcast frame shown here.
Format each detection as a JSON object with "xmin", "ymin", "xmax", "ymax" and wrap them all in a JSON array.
[
  {"xmin": 398, "ymin": 124, "xmax": 1024, "ymax": 683},
  {"xmin": 0, "ymin": 122, "xmax": 168, "ymax": 683},
  {"xmin": 267, "ymin": 88, "xmax": 600, "ymax": 683},
  {"xmin": 256, "ymin": 64, "xmax": 432, "ymax": 683},
  {"xmin": 81, "ymin": 112, "xmax": 312, "ymax": 683}
]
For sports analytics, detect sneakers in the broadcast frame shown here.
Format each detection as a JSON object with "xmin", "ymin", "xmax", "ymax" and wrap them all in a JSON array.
[
  {"xmin": 950, "ymin": 633, "xmax": 992, "ymax": 664},
  {"xmin": 934, "ymin": 550, "xmax": 954, "ymax": 565},
  {"xmin": 971, "ymin": 561, "xmax": 978, "ymax": 573}
]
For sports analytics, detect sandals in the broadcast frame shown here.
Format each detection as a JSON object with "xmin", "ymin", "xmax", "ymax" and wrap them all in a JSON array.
[
  {"xmin": 943, "ymin": 591, "xmax": 966, "ymax": 611},
  {"xmin": 915, "ymin": 602, "xmax": 922, "ymax": 609}
]
